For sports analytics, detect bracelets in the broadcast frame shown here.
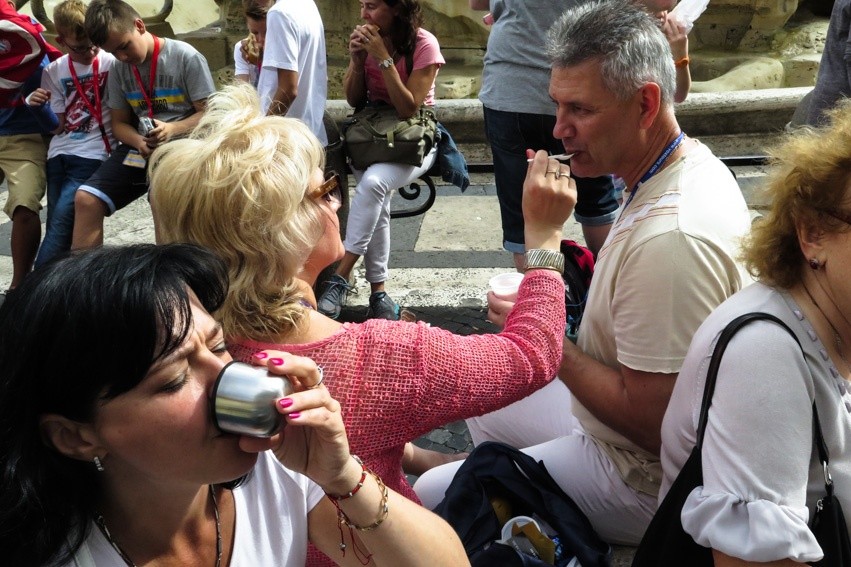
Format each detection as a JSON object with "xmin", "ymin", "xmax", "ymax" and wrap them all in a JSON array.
[
  {"xmin": 321, "ymin": 455, "xmax": 390, "ymax": 566},
  {"xmin": 349, "ymin": 62, "xmax": 364, "ymax": 75},
  {"xmin": 522, "ymin": 249, "xmax": 565, "ymax": 273}
]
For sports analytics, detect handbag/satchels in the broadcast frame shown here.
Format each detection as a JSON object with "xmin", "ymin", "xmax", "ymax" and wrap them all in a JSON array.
[
  {"xmin": 344, "ymin": 103, "xmax": 437, "ymax": 167},
  {"xmin": 637, "ymin": 314, "xmax": 851, "ymax": 567}
]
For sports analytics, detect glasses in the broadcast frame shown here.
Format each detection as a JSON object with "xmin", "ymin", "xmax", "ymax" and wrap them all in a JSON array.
[
  {"xmin": 304, "ymin": 168, "xmax": 345, "ymax": 205},
  {"xmin": 63, "ymin": 39, "xmax": 97, "ymax": 54}
]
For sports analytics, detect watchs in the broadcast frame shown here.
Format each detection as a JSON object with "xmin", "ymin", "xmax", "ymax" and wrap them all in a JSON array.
[{"xmin": 378, "ymin": 58, "xmax": 394, "ymax": 70}]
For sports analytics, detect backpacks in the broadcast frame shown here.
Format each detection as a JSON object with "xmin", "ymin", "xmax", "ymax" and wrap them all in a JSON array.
[{"xmin": 431, "ymin": 440, "xmax": 614, "ymax": 567}]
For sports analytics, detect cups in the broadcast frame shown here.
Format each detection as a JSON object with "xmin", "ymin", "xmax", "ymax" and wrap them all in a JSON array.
[{"xmin": 211, "ymin": 359, "xmax": 296, "ymax": 441}]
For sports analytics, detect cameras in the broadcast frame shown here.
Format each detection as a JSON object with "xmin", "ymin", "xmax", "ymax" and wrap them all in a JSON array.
[{"xmin": 138, "ymin": 116, "xmax": 156, "ymax": 141}]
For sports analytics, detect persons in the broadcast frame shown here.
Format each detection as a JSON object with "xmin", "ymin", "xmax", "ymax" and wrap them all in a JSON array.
[
  {"xmin": 480, "ymin": 0, "xmax": 693, "ymax": 275},
  {"xmin": 0, "ymin": 0, "xmax": 52, "ymax": 293},
  {"xmin": 240, "ymin": 0, "xmax": 328, "ymax": 158},
  {"xmin": 412, "ymin": 2, "xmax": 763, "ymax": 549},
  {"xmin": 24, "ymin": 0, "xmax": 120, "ymax": 274},
  {"xmin": 789, "ymin": 0, "xmax": 851, "ymax": 139},
  {"xmin": 233, "ymin": 0, "xmax": 277, "ymax": 90},
  {"xmin": 659, "ymin": 93, "xmax": 851, "ymax": 567},
  {"xmin": 71, "ymin": 0, "xmax": 219, "ymax": 252},
  {"xmin": 316, "ymin": 0, "xmax": 448, "ymax": 322},
  {"xmin": 2, "ymin": 241, "xmax": 474, "ymax": 566},
  {"xmin": 148, "ymin": 79, "xmax": 580, "ymax": 566}
]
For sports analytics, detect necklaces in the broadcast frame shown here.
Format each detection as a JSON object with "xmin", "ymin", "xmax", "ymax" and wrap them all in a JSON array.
[{"xmin": 91, "ymin": 479, "xmax": 224, "ymax": 567}]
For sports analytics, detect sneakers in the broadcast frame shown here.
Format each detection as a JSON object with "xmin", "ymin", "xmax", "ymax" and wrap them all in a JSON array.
[
  {"xmin": 369, "ymin": 291, "xmax": 399, "ymax": 322},
  {"xmin": 317, "ymin": 274, "xmax": 349, "ymax": 319}
]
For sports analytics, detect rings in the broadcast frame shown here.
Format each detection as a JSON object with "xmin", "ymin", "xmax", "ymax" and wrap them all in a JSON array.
[
  {"xmin": 365, "ymin": 38, "xmax": 369, "ymax": 44},
  {"xmin": 546, "ymin": 168, "xmax": 560, "ymax": 179},
  {"xmin": 559, "ymin": 173, "xmax": 570, "ymax": 180}
]
